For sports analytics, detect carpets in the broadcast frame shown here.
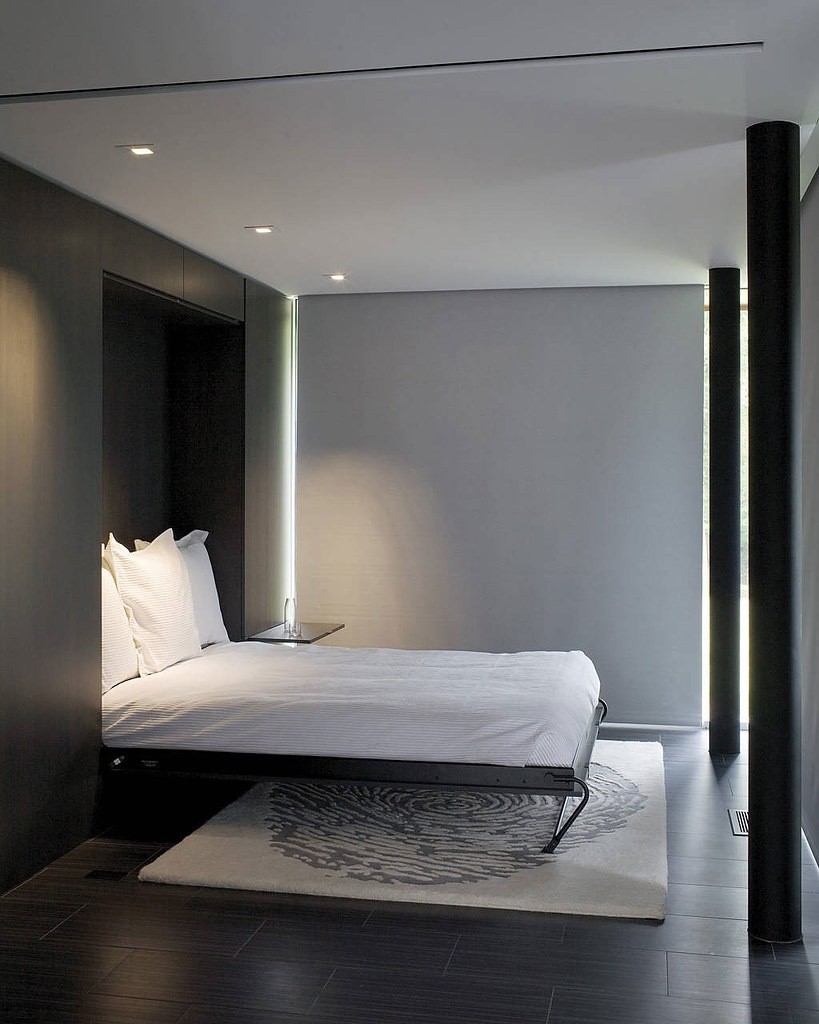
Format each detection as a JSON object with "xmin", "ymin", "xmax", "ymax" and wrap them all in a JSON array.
[{"xmin": 138, "ymin": 738, "xmax": 669, "ymax": 922}]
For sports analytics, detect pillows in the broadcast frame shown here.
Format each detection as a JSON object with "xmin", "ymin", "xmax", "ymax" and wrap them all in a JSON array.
[
  {"xmin": 134, "ymin": 530, "xmax": 229, "ymax": 649},
  {"xmin": 105, "ymin": 528, "xmax": 204, "ymax": 678},
  {"xmin": 100, "ymin": 543, "xmax": 140, "ymax": 695}
]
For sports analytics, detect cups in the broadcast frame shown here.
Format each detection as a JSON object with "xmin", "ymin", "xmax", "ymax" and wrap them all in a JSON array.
[{"xmin": 289, "ymin": 623, "xmax": 301, "ymax": 637}]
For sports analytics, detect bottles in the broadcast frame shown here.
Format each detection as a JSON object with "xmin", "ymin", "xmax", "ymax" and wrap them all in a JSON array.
[{"xmin": 284, "ymin": 598, "xmax": 296, "ymax": 632}]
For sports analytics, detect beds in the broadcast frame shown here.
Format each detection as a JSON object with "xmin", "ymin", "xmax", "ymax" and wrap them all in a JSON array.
[{"xmin": 98, "ymin": 527, "xmax": 607, "ymax": 856}]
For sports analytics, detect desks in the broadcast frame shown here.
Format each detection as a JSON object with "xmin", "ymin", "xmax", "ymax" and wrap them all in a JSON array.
[{"xmin": 248, "ymin": 621, "xmax": 344, "ymax": 644}]
